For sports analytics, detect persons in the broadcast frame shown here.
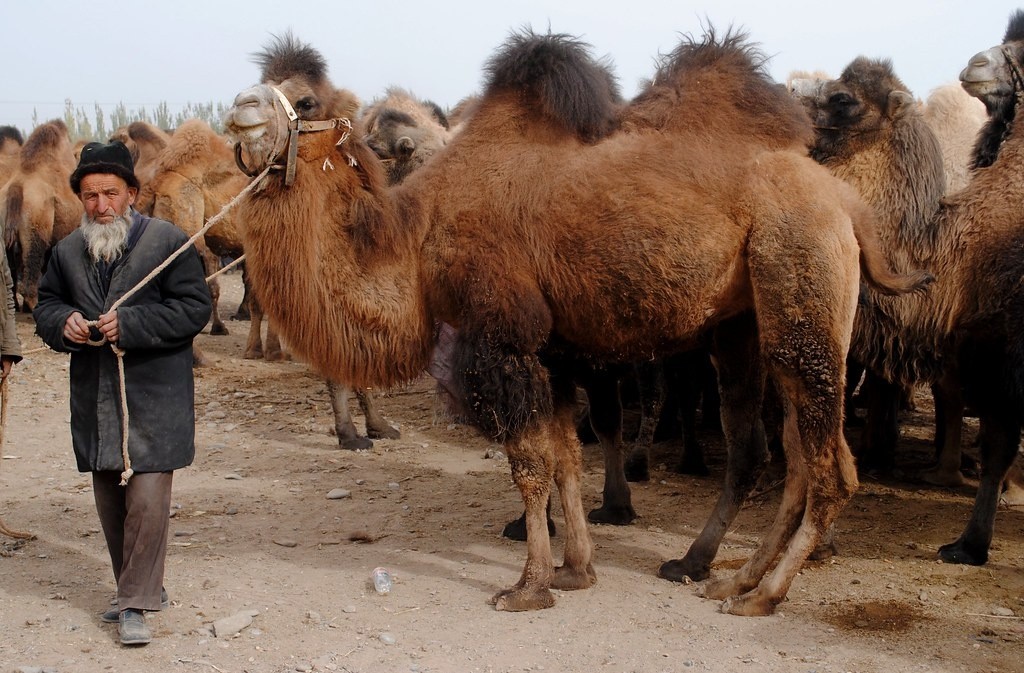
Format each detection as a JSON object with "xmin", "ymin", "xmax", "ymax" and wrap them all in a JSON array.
[
  {"xmin": 33, "ymin": 140, "xmax": 217, "ymax": 648},
  {"xmin": 0, "ymin": 230, "xmax": 23, "ymax": 381}
]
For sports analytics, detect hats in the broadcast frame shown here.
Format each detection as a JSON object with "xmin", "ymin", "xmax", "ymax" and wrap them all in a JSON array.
[{"xmin": 70, "ymin": 141, "xmax": 140, "ymax": 194}]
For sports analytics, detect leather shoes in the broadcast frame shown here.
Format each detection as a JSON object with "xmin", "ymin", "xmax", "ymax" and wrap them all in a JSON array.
[
  {"xmin": 117, "ymin": 609, "xmax": 151, "ymax": 644},
  {"xmin": 102, "ymin": 587, "xmax": 169, "ymax": 622}
]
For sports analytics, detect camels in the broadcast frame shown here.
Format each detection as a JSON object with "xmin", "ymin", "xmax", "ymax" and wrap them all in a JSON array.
[{"xmin": 1, "ymin": 10, "xmax": 1024, "ymax": 617}]
